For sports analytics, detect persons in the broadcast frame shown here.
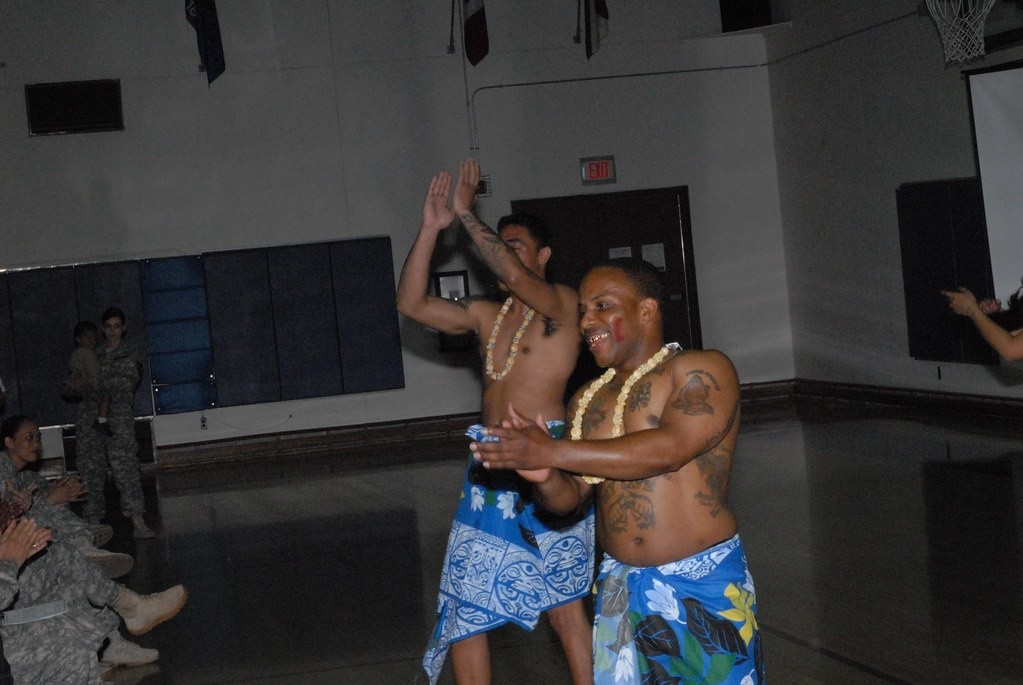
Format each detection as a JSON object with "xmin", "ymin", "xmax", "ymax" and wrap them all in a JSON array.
[
  {"xmin": 395, "ymin": 158, "xmax": 596, "ymax": 685},
  {"xmin": 58, "ymin": 309, "xmax": 155, "ymax": 539},
  {"xmin": 470, "ymin": 258, "xmax": 767, "ymax": 685},
  {"xmin": 940, "ymin": 286, "xmax": 1023, "ymax": 362},
  {"xmin": 0, "ymin": 415, "xmax": 189, "ymax": 685}
]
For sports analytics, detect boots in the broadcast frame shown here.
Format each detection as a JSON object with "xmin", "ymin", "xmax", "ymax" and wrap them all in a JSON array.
[
  {"xmin": 98, "ymin": 630, "xmax": 160, "ymax": 665},
  {"xmin": 113, "ymin": 584, "xmax": 188, "ymax": 635}
]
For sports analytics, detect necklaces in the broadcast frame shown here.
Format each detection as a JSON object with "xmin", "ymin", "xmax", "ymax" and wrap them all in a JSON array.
[
  {"xmin": 484, "ymin": 297, "xmax": 535, "ymax": 382},
  {"xmin": 569, "ymin": 341, "xmax": 683, "ymax": 485}
]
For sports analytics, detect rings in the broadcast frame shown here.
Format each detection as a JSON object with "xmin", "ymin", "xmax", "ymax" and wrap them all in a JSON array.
[
  {"xmin": 11, "ymin": 515, "xmax": 14, "ymax": 518},
  {"xmin": 33, "ymin": 543, "xmax": 36, "ymax": 547}
]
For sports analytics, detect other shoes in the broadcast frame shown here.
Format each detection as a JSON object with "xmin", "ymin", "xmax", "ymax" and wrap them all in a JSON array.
[
  {"xmin": 70, "ymin": 537, "xmax": 134, "ymax": 579},
  {"xmin": 92, "ymin": 419, "xmax": 113, "ymax": 437},
  {"xmin": 88, "ymin": 524, "xmax": 114, "ymax": 547},
  {"xmin": 131, "ymin": 515, "xmax": 156, "ymax": 539}
]
